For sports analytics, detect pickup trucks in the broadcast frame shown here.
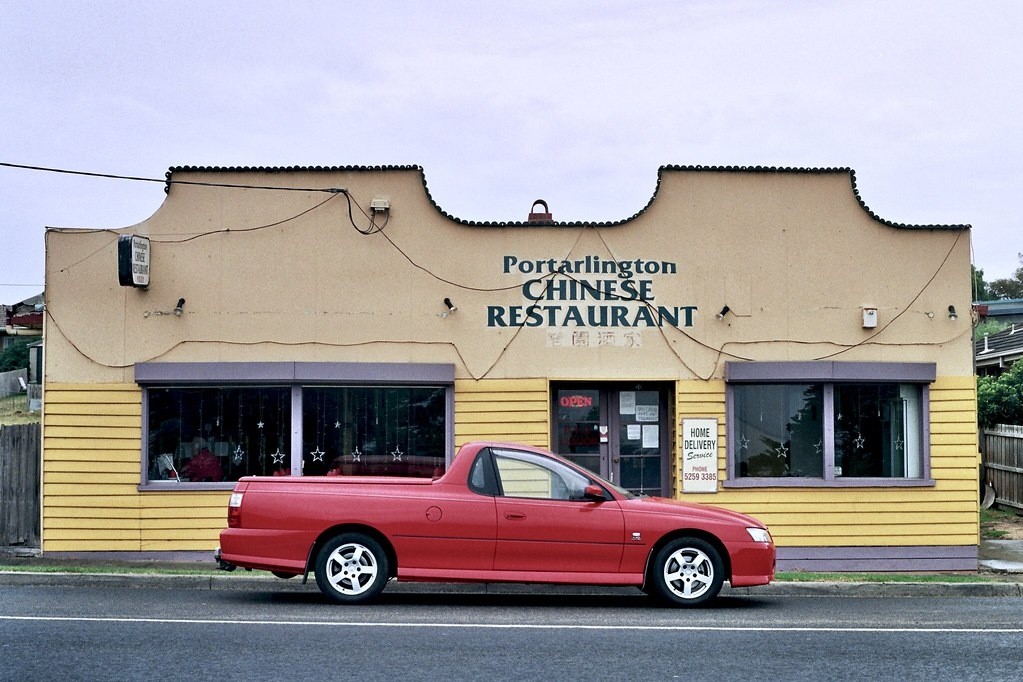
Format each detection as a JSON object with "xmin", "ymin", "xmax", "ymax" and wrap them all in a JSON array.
[{"xmin": 214, "ymin": 437, "xmax": 778, "ymax": 605}]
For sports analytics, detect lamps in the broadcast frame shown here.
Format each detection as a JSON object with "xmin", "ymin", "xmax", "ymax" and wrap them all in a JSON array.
[
  {"xmin": 948, "ymin": 306, "xmax": 958, "ymax": 321},
  {"xmin": 444, "ymin": 298, "xmax": 459, "ymax": 313},
  {"xmin": 174, "ymin": 298, "xmax": 185, "ymax": 315},
  {"xmin": 715, "ymin": 306, "xmax": 730, "ymax": 321}
]
